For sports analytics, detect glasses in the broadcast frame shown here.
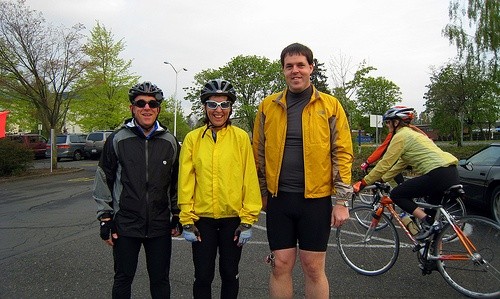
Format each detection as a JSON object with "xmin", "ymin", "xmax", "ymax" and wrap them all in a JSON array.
[
  {"xmin": 385, "ymin": 121, "xmax": 391, "ymax": 124},
  {"xmin": 206, "ymin": 99, "xmax": 232, "ymax": 110},
  {"xmin": 131, "ymin": 99, "xmax": 161, "ymax": 108}
]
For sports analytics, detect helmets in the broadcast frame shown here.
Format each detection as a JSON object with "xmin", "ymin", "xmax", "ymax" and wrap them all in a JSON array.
[
  {"xmin": 383, "ymin": 106, "xmax": 418, "ymax": 124},
  {"xmin": 199, "ymin": 79, "xmax": 237, "ymax": 105},
  {"xmin": 129, "ymin": 81, "xmax": 163, "ymax": 103}
]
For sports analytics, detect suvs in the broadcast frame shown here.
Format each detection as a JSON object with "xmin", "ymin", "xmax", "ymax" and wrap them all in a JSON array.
[
  {"xmin": 6, "ymin": 132, "xmax": 48, "ymax": 160},
  {"xmin": 45, "ymin": 133, "xmax": 89, "ymax": 160},
  {"xmin": 85, "ymin": 130, "xmax": 116, "ymax": 158}
]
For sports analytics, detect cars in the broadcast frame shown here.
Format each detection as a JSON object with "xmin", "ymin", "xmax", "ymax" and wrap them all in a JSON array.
[{"xmin": 456, "ymin": 142, "xmax": 499, "ymax": 227}]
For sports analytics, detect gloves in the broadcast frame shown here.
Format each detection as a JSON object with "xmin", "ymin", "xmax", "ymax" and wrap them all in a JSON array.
[
  {"xmin": 360, "ymin": 162, "xmax": 369, "ymax": 171},
  {"xmin": 352, "ymin": 181, "xmax": 367, "ymax": 197}
]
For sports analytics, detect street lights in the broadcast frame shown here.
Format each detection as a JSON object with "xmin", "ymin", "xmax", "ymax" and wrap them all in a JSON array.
[{"xmin": 164, "ymin": 61, "xmax": 187, "ymax": 137}]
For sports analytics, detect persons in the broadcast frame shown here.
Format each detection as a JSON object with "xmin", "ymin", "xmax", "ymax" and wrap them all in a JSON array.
[
  {"xmin": 252, "ymin": 43, "xmax": 354, "ymax": 299},
  {"xmin": 177, "ymin": 79, "xmax": 263, "ymax": 299},
  {"xmin": 91, "ymin": 80, "xmax": 180, "ymax": 299},
  {"xmin": 360, "ymin": 105, "xmax": 430, "ymax": 217},
  {"xmin": 352, "ymin": 107, "xmax": 460, "ymax": 271}
]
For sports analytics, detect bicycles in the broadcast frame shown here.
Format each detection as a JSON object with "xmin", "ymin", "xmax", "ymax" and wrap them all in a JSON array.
[
  {"xmin": 351, "ymin": 164, "xmax": 466, "ymax": 242},
  {"xmin": 335, "ymin": 180, "xmax": 500, "ymax": 299}
]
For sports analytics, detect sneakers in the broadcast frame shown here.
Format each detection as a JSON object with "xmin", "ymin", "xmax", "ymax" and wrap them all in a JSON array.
[
  {"xmin": 414, "ymin": 214, "xmax": 443, "ymax": 241},
  {"xmin": 418, "ymin": 254, "xmax": 446, "ymax": 269}
]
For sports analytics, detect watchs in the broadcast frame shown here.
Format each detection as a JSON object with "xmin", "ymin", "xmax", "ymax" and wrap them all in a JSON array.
[{"xmin": 336, "ymin": 201, "xmax": 349, "ymax": 206}]
